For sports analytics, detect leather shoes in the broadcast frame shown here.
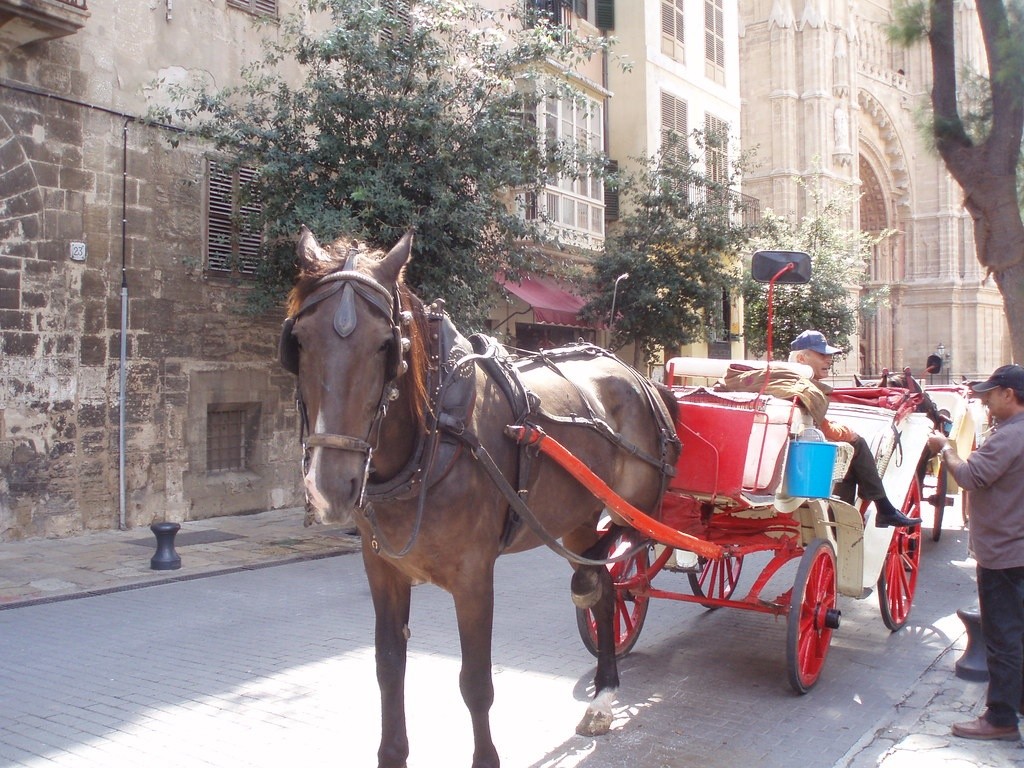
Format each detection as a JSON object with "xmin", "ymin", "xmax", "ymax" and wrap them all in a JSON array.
[
  {"xmin": 875, "ymin": 509, "xmax": 923, "ymax": 528},
  {"xmin": 952, "ymin": 715, "xmax": 1021, "ymax": 741}
]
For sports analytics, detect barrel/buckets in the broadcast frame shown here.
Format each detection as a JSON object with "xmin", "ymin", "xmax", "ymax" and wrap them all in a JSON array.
[{"xmin": 788, "ymin": 429, "xmax": 838, "ymax": 502}]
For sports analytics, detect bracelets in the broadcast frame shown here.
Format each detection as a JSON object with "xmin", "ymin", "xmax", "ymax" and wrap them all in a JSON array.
[{"xmin": 937, "ymin": 446, "xmax": 954, "ymax": 463}]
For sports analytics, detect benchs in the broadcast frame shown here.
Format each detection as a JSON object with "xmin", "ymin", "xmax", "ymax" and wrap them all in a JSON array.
[
  {"xmin": 824, "ymin": 410, "xmax": 887, "ymax": 500},
  {"xmin": 927, "ymin": 392, "xmax": 963, "ymax": 427},
  {"xmin": 663, "ymin": 356, "xmax": 814, "ymax": 486}
]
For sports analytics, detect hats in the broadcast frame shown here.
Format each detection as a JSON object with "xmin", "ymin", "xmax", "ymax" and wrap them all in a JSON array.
[
  {"xmin": 971, "ymin": 364, "xmax": 1024, "ymax": 392},
  {"xmin": 790, "ymin": 330, "xmax": 843, "ymax": 355}
]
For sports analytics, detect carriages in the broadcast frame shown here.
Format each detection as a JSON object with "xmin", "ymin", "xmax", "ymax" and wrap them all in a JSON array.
[
  {"xmin": 277, "ymin": 224, "xmax": 934, "ymax": 768},
  {"xmin": 853, "ymin": 368, "xmax": 990, "ymax": 537}
]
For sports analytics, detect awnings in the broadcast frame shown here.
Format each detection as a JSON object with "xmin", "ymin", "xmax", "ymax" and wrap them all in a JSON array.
[{"xmin": 478, "ymin": 258, "xmax": 625, "ymax": 331}]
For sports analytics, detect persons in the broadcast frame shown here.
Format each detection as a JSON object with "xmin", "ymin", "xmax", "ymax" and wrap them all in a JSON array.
[
  {"xmin": 927, "ymin": 363, "xmax": 1024, "ymax": 741},
  {"xmin": 787, "ymin": 329, "xmax": 923, "ymax": 528}
]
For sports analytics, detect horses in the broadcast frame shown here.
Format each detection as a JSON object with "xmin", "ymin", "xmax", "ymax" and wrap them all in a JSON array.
[{"xmin": 288, "ymin": 222, "xmax": 679, "ymax": 768}]
